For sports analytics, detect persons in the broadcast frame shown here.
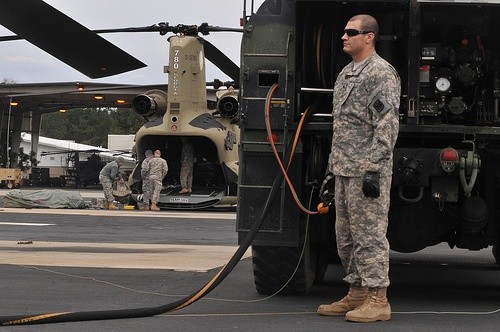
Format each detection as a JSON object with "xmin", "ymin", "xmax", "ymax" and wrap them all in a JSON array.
[
  {"xmin": 147, "ymin": 150, "xmax": 168, "ymax": 210},
  {"xmin": 99, "ymin": 156, "xmax": 124, "ymax": 209},
  {"xmin": 139, "ymin": 150, "xmax": 154, "ymax": 209},
  {"xmin": 113, "ymin": 173, "xmax": 127, "ymax": 201},
  {"xmin": 318, "ymin": 14, "xmax": 402, "ymax": 322},
  {"xmin": 179, "ymin": 136, "xmax": 194, "ymax": 193}
]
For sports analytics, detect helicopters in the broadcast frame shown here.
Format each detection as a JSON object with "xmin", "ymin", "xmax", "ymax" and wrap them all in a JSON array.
[{"xmin": 0, "ymin": 22, "xmax": 244, "ymax": 210}]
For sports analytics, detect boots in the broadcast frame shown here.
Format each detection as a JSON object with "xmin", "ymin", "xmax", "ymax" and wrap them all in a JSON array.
[
  {"xmin": 345, "ymin": 287, "xmax": 391, "ymax": 322},
  {"xmin": 144, "ymin": 204, "xmax": 150, "ymax": 210},
  {"xmin": 186, "ymin": 188, "xmax": 192, "ymax": 195},
  {"xmin": 317, "ymin": 286, "xmax": 368, "ymax": 317},
  {"xmin": 105, "ymin": 201, "xmax": 109, "ymax": 209},
  {"xmin": 179, "ymin": 187, "xmax": 188, "ymax": 194},
  {"xmin": 108, "ymin": 201, "xmax": 119, "ymax": 210},
  {"xmin": 150, "ymin": 203, "xmax": 160, "ymax": 211}
]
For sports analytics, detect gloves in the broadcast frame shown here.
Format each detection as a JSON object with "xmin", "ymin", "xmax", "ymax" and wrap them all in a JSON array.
[
  {"xmin": 362, "ymin": 170, "xmax": 380, "ymax": 198},
  {"xmin": 323, "ymin": 172, "xmax": 337, "ymax": 202}
]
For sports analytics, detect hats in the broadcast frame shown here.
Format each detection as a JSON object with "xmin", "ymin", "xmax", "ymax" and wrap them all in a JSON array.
[{"xmin": 115, "ymin": 157, "xmax": 125, "ymax": 163}]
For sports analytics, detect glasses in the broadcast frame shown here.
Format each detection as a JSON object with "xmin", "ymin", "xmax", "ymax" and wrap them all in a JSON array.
[{"xmin": 342, "ymin": 28, "xmax": 375, "ymax": 37}]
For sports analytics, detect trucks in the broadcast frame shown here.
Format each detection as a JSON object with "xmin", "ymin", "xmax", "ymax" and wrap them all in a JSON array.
[{"xmin": 235, "ymin": 0, "xmax": 500, "ymax": 297}]
